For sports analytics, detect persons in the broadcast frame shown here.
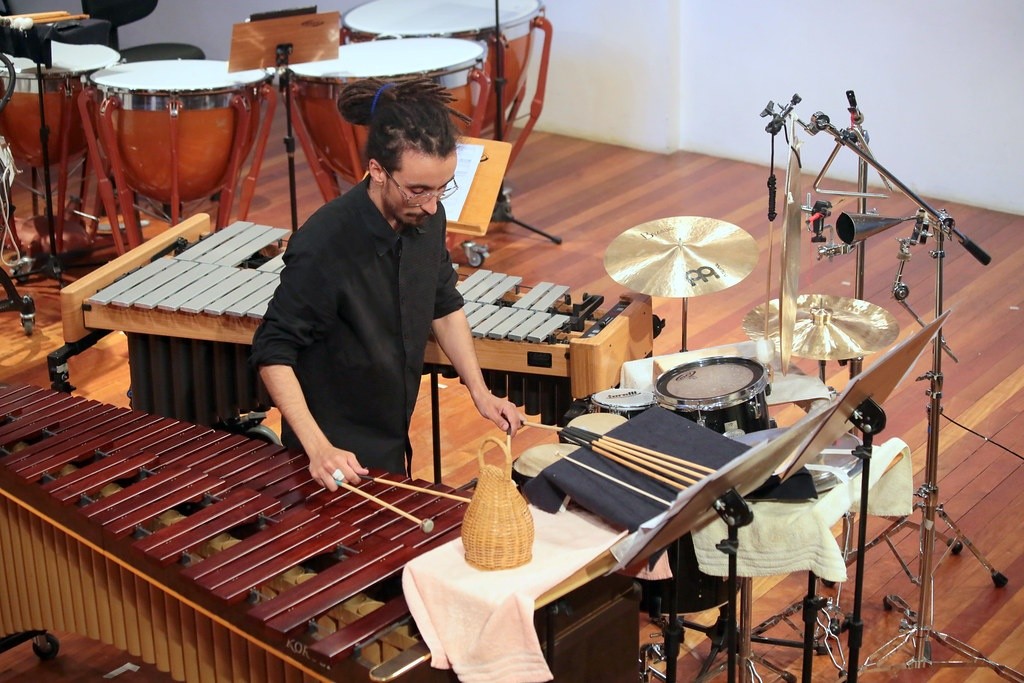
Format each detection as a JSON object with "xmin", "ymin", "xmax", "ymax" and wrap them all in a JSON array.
[{"xmin": 251, "ymin": 77, "xmax": 520, "ymax": 604}]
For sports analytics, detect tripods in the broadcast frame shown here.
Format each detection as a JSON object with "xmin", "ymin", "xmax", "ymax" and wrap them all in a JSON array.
[{"xmin": 648, "ymin": 92, "xmax": 1024, "ymax": 683}]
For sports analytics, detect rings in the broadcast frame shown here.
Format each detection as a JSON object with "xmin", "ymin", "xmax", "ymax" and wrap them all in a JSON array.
[{"xmin": 332, "ymin": 469, "xmax": 344, "ymax": 481}]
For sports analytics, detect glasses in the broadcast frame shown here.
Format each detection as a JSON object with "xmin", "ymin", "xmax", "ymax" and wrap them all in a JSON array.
[{"xmin": 380, "ymin": 166, "xmax": 458, "ymax": 206}]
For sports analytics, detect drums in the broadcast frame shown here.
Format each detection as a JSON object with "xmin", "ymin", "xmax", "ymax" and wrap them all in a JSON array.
[
  {"xmin": 587, "ymin": 388, "xmax": 656, "ymax": 421},
  {"xmin": 0, "ymin": 40, "xmax": 276, "ymax": 202},
  {"xmin": 510, "ymin": 443, "xmax": 581, "ymax": 488},
  {"xmin": 620, "ymin": 531, "xmax": 744, "ymax": 614},
  {"xmin": 281, "ymin": 0, "xmax": 544, "ymax": 186},
  {"xmin": 558, "ymin": 411, "xmax": 628, "ymax": 444},
  {"xmin": 651, "ymin": 355, "xmax": 778, "ymax": 437}
]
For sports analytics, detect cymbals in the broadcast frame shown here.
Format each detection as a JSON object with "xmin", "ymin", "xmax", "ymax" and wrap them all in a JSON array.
[
  {"xmin": 741, "ymin": 294, "xmax": 900, "ymax": 361},
  {"xmin": 601, "ymin": 216, "xmax": 761, "ymax": 298}
]
